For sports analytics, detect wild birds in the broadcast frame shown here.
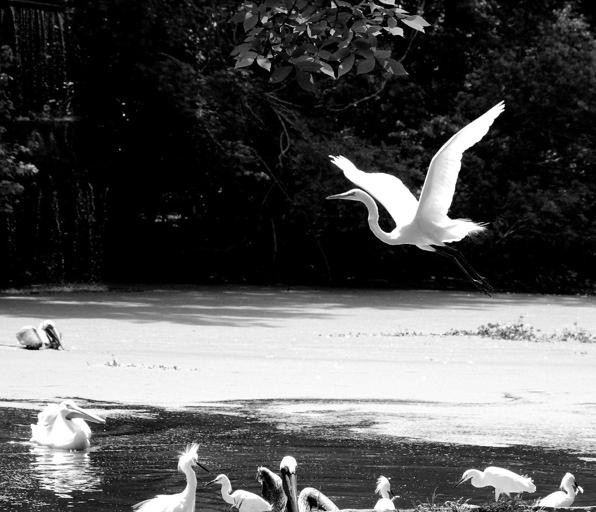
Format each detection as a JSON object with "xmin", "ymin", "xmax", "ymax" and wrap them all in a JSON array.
[
  {"xmin": 372, "ymin": 474, "xmax": 396, "ymax": 512},
  {"xmin": 28, "ymin": 399, "xmax": 108, "ymax": 451},
  {"xmin": 278, "ymin": 454, "xmax": 342, "ymax": 512},
  {"xmin": 254, "ymin": 466, "xmax": 289, "ymax": 512},
  {"xmin": 129, "ymin": 441, "xmax": 210, "ymax": 512},
  {"xmin": 535, "ymin": 472, "xmax": 584, "ymax": 508},
  {"xmin": 323, "ymin": 98, "xmax": 509, "ymax": 255},
  {"xmin": 204, "ymin": 473, "xmax": 275, "ymax": 512},
  {"xmin": 453, "ymin": 466, "xmax": 537, "ymax": 502}
]
